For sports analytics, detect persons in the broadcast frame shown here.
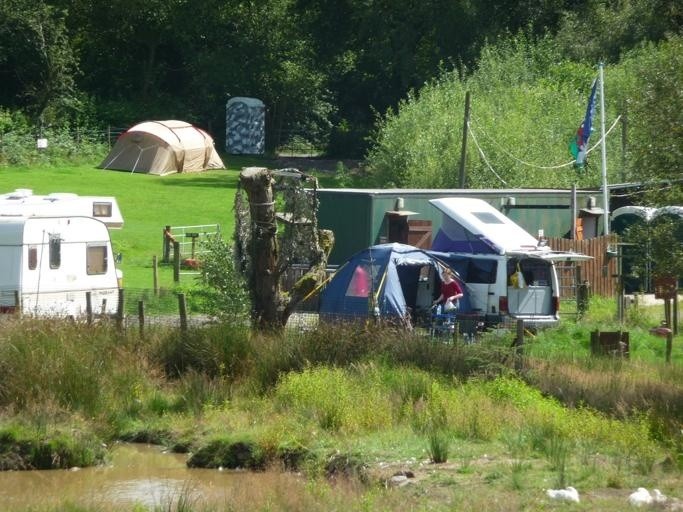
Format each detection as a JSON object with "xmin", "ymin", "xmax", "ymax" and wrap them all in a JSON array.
[
  {"xmin": 575, "ymin": 279, "xmax": 589, "ymax": 316},
  {"xmin": 427, "ymin": 267, "xmax": 463, "ymax": 312}
]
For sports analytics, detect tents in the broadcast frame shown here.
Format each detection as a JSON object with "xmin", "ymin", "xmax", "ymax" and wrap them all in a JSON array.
[
  {"xmin": 311, "ymin": 240, "xmax": 476, "ymax": 334},
  {"xmin": 91, "ymin": 117, "xmax": 228, "ymax": 175}
]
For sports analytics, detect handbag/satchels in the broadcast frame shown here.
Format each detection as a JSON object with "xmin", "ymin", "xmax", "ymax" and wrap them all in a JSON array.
[{"xmin": 510, "ymin": 263, "xmax": 525, "ymax": 288}]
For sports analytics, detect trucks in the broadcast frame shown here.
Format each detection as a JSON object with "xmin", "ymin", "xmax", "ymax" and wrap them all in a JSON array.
[{"xmin": 0, "ymin": 191, "xmax": 124, "ymax": 327}]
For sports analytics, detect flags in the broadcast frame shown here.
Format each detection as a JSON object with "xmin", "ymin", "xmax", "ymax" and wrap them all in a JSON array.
[{"xmin": 566, "ymin": 75, "xmax": 600, "ymax": 180}]
